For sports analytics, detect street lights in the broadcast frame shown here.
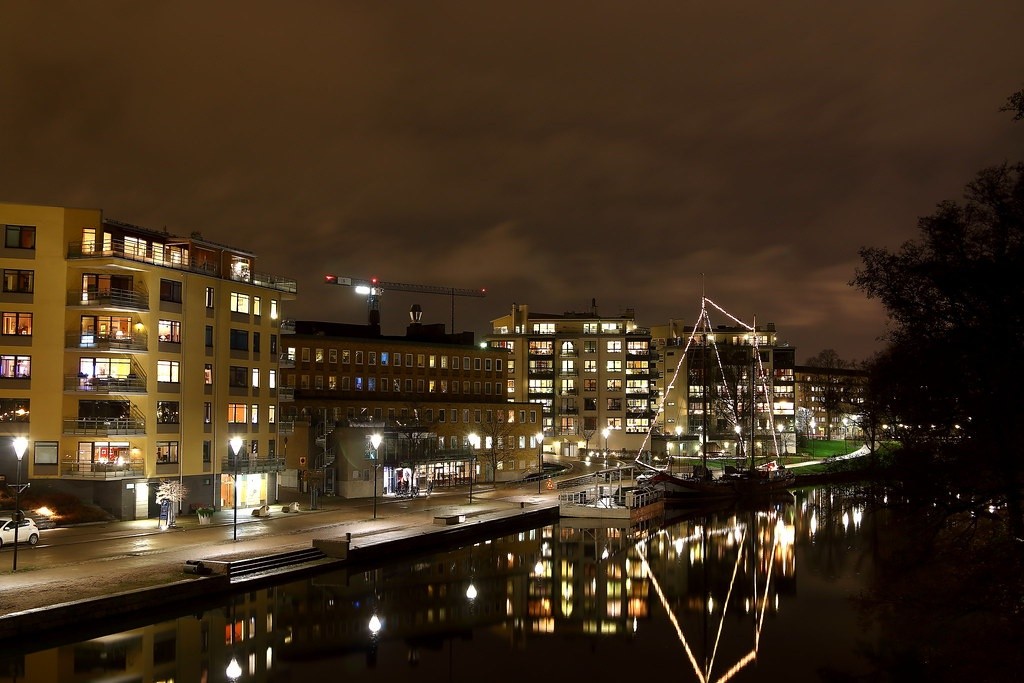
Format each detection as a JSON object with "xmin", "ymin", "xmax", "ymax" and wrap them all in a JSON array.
[
  {"xmin": 602, "ymin": 429, "xmax": 610, "ymax": 483},
  {"xmin": 536, "ymin": 433, "xmax": 544, "ymax": 495},
  {"xmin": 371, "ymin": 433, "xmax": 382, "ymax": 519},
  {"xmin": 675, "ymin": 426, "xmax": 683, "ymax": 473},
  {"xmin": 12, "ymin": 437, "xmax": 28, "ymax": 572},
  {"xmin": 810, "ymin": 421, "xmax": 815, "ymax": 460},
  {"xmin": 842, "ymin": 418, "xmax": 848, "ymax": 453},
  {"xmin": 777, "ymin": 424, "xmax": 784, "ymax": 465},
  {"xmin": 467, "ymin": 433, "xmax": 478, "ymax": 504},
  {"xmin": 230, "ymin": 436, "xmax": 243, "ymax": 542}
]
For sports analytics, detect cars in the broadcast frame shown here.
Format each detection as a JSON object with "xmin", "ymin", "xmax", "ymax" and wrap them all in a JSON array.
[{"xmin": 0, "ymin": 517, "xmax": 39, "ymax": 548}]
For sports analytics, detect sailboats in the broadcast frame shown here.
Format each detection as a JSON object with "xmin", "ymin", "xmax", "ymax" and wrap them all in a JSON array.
[{"xmin": 635, "ymin": 272, "xmax": 796, "ymax": 503}]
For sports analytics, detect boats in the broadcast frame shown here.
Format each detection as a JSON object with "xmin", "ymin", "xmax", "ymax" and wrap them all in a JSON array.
[{"xmin": 557, "ymin": 472, "xmax": 665, "ymax": 520}]
